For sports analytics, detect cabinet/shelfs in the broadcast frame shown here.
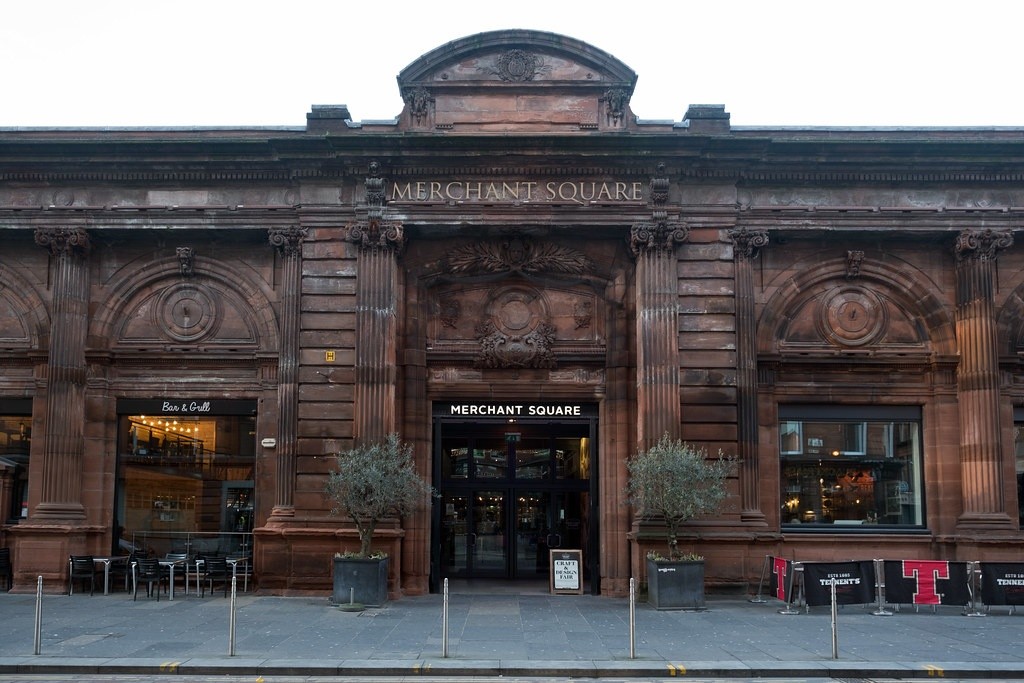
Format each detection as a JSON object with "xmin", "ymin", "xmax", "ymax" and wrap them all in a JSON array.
[{"xmin": 124, "ymin": 492, "xmax": 197, "ymax": 530}]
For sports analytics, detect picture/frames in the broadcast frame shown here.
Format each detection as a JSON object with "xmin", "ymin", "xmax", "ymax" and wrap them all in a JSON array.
[{"xmin": 549, "ymin": 549, "xmax": 583, "ymax": 595}]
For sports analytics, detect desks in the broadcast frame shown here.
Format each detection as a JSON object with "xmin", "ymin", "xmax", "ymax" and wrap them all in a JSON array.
[
  {"xmin": 196, "ymin": 557, "xmax": 248, "ymax": 599},
  {"xmin": 69, "ymin": 555, "xmax": 128, "ymax": 597},
  {"xmin": 131, "ymin": 559, "xmax": 189, "ymax": 601}
]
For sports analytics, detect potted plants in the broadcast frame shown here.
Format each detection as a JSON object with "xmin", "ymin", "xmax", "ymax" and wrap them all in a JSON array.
[
  {"xmin": 322, "ymin": 432, "xmax": 439, "ymax": 608},
  {"xmin": 623, "ymin": 430, "xmax": 743, "ymax": 610}
]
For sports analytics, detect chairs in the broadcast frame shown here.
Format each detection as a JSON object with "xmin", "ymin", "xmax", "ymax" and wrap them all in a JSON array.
[
  {"xmin": 157, "ymin": 554, "xmax": 187, "ymax": 597},
  {"xmin": 202, "ymin": 557, "xmax": 227, "ymax": 599},
  {"xmin": 95, "ymin": 553, "xmax": 130, "ymax": 592},
  {"xmin": 0, "ymin": 547, "xmax": 13, "ymax": 593},
  {"xmin": 133, "ymin": 558, "xmax": 175, "ymax": 602},
  {"xmin": 68, "ymin": 555, "xmax": 103, "ymax": 597}
]
